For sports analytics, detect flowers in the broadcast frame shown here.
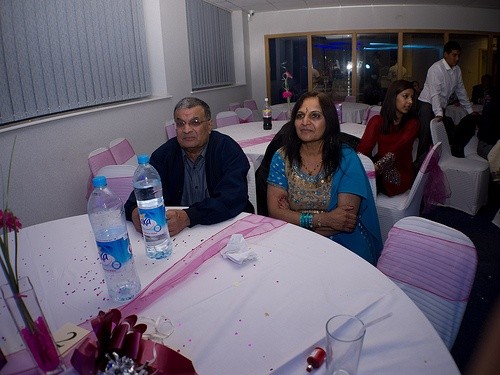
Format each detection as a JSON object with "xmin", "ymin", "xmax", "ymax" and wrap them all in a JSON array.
[
  {"xmin": 282, "ymin": 72, "xmax": 293, "ymax": 109},
  {"xmin": 0, "ymin": 135, "xmax": 24, "ymax": 295}
]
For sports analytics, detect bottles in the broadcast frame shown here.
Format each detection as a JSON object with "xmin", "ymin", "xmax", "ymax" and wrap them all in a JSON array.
[
  {"xmin": 263, "ymin": 97, "xmax": 273, "ymax": 130},
  {"xmin": 132, "ymin": 153, "xmax": 173, "ymax": 259},
  {"xmin": 87, "ymin": 176, "xmax": 141, "ymax": 305},
  {"xmin": 306, "ymin": 346, "xmax": 326, "ymax": 373}
]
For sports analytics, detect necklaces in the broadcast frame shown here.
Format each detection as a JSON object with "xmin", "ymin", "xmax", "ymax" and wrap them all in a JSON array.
[{"xmin": 302, "ymin": 161, "xmax": 322, "ymax": 176}]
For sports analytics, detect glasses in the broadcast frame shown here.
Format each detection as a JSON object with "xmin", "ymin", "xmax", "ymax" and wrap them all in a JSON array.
[{"xmin": 177, "ymin": 119, "xmax": 209, "ymax": 127}]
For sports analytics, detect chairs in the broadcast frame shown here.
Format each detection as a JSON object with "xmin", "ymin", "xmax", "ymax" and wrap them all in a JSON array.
[
  {"xmin": 85, "ymin": 137, "xmax": 140, "ymax": 207},
  {"xmin": 375, "ymin": 215, "xmax": 477, "ymax": 353},
  {"xmin": 164, "ymin": 99, "xmax": 500, "ymax": 243}
]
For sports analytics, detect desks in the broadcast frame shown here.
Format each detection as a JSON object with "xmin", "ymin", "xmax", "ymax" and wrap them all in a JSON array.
[
  {"xmin": 0, "ymin": 199, "xmax": 463, "ymax": 375},
  {"xmin": 213, "ymin": 120, "xmax": 290, "ymax": 215}
]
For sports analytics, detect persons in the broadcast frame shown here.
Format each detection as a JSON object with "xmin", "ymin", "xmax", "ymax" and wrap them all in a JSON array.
[
  {"xmin": 472, "ymin": 74, "xmax": 495, "ymax": 104},
  {"xmin": 416, "ymin": 41, "xmax": 479, "ymax": 164},
  {"xmin": 356, "ymin": 80, "xmax": 421, "ymax": 197},
  {"xmin": 264, "ymin": 91, "xmax": 386, "ymax": 265},
  {"xmin": 124, "ymin": 97, "xmax": 255, "ymax": 238},
  {"xmin": 412, "ymin": 81, "xmax": 421, "ymax": 111}
]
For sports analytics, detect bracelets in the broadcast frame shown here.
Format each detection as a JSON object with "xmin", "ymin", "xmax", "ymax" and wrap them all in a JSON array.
[
  {"xmin": 313, "ymin": 214, "xmax": 322, "ymax": 229},
  {"xmin": 299, "ymin": 213, "xmax": 313, "ymax": 229}
]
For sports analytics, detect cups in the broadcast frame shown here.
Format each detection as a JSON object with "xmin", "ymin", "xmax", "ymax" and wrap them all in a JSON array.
[
  {"xmin": 326, "ymin": 314, "xmax": 366, "ymax": 375},
  {"xmin": 335, "ymin": 104, "xmax": 342, "ymax": 124}
]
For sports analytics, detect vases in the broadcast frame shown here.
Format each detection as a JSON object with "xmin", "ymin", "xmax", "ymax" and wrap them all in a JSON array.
[{"xmin": 0, "ymin": 276, "xmax": 68, "ymax": 375}]
